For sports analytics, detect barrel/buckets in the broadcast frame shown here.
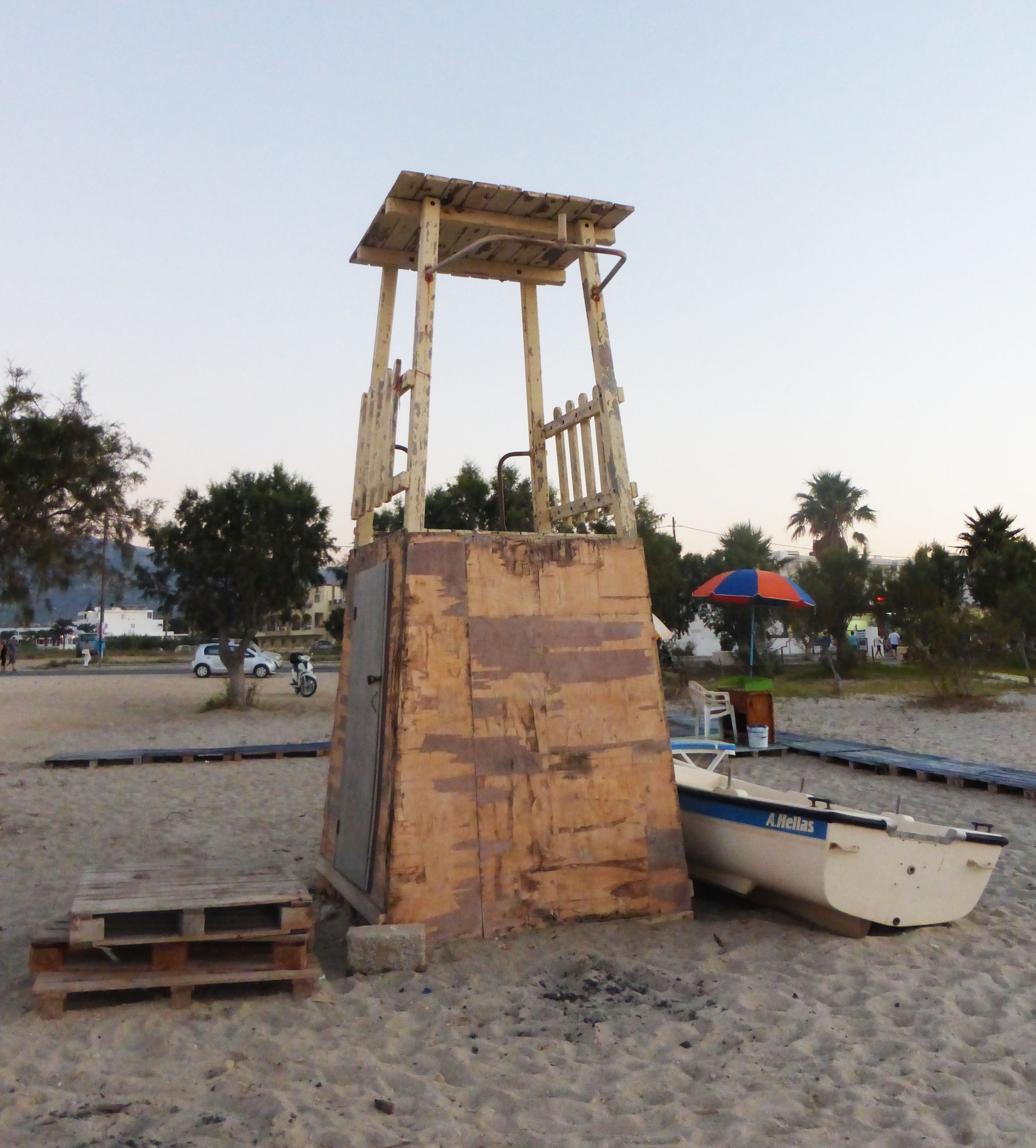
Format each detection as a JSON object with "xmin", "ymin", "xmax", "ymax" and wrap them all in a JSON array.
[{"xmin": 747, "ymin": 724, "xmax": 769, "ymax": 748}]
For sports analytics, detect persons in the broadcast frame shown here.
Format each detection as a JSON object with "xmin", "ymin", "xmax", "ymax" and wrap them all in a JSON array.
[
  {"xmin": 3, "ymin": 636, "xmax": 20, "ymax": 672},
  {"xmin": 94, "ymin": 634, "xmax": 107, "ymax": 667},
  {"xmin": 873, "ymin": 632, "xmax": 885, "ymax": 658},
  {"xmin": 0, "ymin": 637, "xmax": 13, "ymax": 672},
  {"xmin": 848, "ymin": 630, "xmax": 859, "ymax": 649},
  {"xmin": 888, "ymin": 629, "xmax": 901, "ymax": 660},
  {"xmin": 821, "ymin": 631, "xmax": 832, "ymax": 651},
  {"xmin": 81, "ymin": 639, "xmax": 92, "ymax": 667}
]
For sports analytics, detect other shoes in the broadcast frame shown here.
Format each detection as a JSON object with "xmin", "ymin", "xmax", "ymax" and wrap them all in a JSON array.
[
  {"xmin": 12, "ymin": 669, "xmax": 18, "ymax": 672},
  {"xmin": 84, "ymin": 665, "xmax": 89, "ymax": 668}
]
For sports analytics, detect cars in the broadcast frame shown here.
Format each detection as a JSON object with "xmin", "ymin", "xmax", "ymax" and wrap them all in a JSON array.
[{"xmin": 191, "ymin": 637, "xmax": 284, "ymax": 678}]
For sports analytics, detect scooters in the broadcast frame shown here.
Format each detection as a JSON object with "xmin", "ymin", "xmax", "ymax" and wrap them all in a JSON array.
[{"xmin": 289, "ymin": 652, "xmax": 317, "ymax": 697}]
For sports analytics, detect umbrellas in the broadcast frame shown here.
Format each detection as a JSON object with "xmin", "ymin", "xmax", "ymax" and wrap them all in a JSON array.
[{"xmin": 689, "ymin": 562, "xmax": 818, "ymax": 664}]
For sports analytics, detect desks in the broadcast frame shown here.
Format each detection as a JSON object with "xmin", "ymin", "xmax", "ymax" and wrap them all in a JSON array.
[{"xmin": 712, "ymin": 685, "xmax": 778, "ymax": 744}]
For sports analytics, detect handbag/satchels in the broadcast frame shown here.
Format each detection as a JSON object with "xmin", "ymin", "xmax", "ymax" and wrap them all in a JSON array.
[{"xmin": 82, "ymin": 649, "xmax": 89, "ymax": 655}]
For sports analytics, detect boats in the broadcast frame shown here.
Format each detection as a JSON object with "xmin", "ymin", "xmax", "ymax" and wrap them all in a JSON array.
[{"xmin": 668, "ymin": 735, "xmax": 1006, "ymax": 941}]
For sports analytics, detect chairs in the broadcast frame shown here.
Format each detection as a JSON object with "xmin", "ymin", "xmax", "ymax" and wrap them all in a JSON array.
[{"xmin": 687, "ymin": 682, "xmax": 741, "ymax": 746}]
